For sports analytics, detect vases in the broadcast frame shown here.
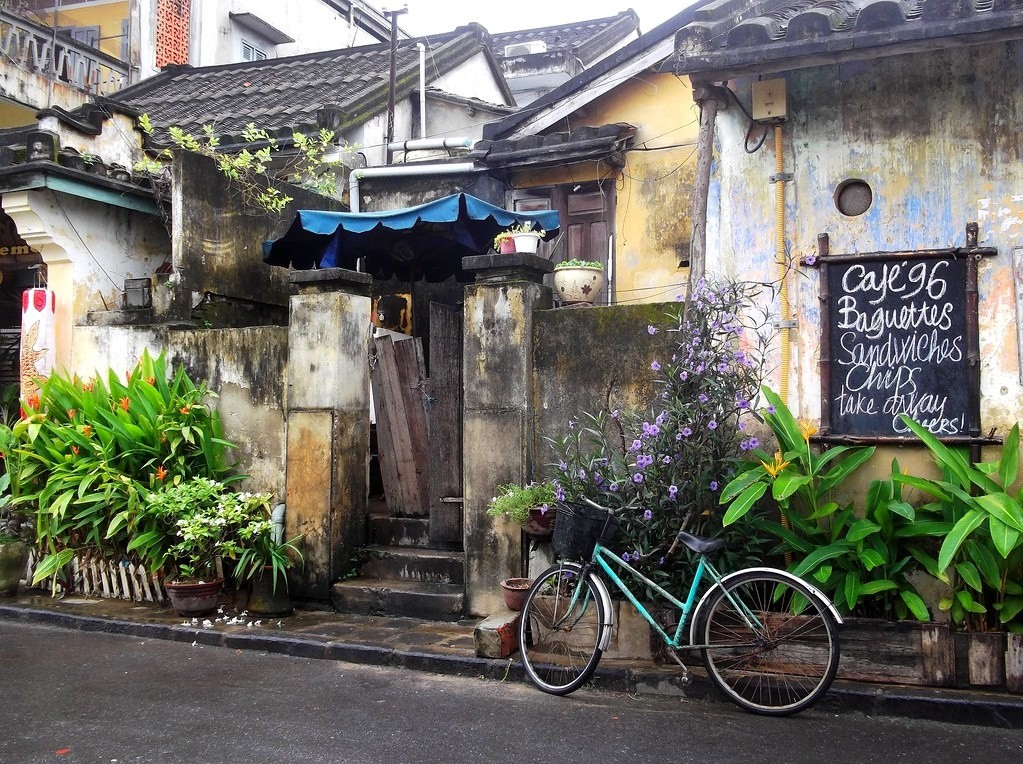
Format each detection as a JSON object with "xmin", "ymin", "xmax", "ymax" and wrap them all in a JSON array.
[
  {"xmin": 520, "ymin": 508, "xmax": 557, "ymax": 541},
  {"xmin": 500, "ymin": 575, "xmax": 533, "ymax": 611},
  {"xmin": 162, "ymin": 575, "xmax": 226, "ymax": 616}
]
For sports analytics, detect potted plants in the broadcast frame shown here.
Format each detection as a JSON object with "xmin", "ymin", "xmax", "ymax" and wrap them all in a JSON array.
[
  {"xmin": 493, "ymin": 221, "xmax": 546, "ymax": 253},
  {"xmin": 231, "ymin": 526, "xmax": 305, "ymax": 618},
  {"xmin": 554, "ymin": 258, "xmax": 603, "ymax": 306}
]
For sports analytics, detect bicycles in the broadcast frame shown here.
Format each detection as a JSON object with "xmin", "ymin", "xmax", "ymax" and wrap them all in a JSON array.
[{"xmin": 516, "ymin": 490, "xmax": 846, "ymax": 717}]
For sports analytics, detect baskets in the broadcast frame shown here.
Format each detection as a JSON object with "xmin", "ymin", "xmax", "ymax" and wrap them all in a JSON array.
[{"xmin": 552, "ymin": 500, "xmax": 621, "ymax": 560}]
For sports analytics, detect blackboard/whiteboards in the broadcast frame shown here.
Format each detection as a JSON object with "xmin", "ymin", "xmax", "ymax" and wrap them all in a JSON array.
[{"xmin": 817, "ymin": 246, "xmax": 984, "ymax": 446}]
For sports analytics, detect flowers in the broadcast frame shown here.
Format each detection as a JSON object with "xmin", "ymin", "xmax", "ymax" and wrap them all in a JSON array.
[
  {"xmin": 485, "ymin": 482, "xmax": 557, "ymax": 523},
  {"xmin": 146, "ymin": 475, "xmax": 275, "ymax": 578}
]
